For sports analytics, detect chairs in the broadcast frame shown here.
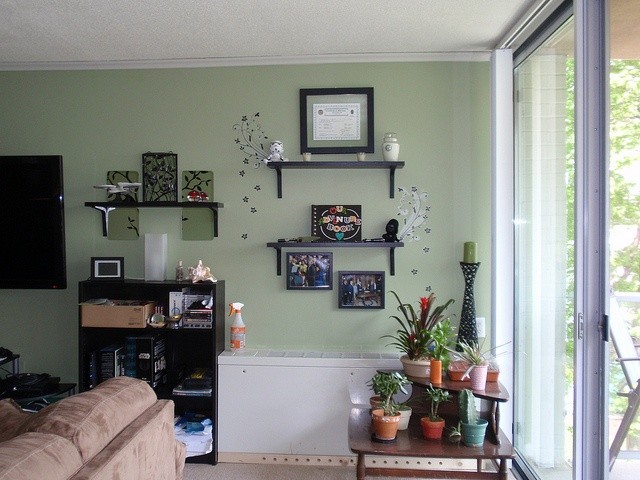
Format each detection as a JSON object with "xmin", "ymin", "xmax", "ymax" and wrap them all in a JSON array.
[{"xmin": 607, "ymin": 290, "xmax": 640, "ymax": 476}]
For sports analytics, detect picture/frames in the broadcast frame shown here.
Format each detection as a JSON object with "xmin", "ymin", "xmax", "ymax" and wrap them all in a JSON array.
[
  {"xmin": 285, "ymin": 251, "xmax": 334, "ymax": 290},
  {"xmin": 299, "ymin": 86, "xmax": 374, "ymax": 154},
  {"xmin": 337, "ymin": 270, "xmax": 385, "ymax": 309}
]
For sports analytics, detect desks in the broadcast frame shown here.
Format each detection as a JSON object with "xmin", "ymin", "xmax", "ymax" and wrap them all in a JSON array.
[
  {"xmin": 0, "ymin": 353, "xmax": 20, "ymax": 378},
  {"xmin": 347, "ymin": 407, "xmax": 517, "ymax": 480},
  {"xmin": 401, "ymin": 370, "xmax": 509, "ymax": 445}
]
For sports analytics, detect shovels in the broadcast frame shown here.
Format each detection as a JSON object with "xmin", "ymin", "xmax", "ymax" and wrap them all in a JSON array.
[{"xmin": 277, "ymin": 236, "xmax": 321, "ymax": 243}]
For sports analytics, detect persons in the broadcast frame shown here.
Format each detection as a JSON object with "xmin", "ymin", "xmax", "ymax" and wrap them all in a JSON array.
[
  {"xmin": 287, "ymin": 255, "xmax": 330, "ymax": 287},
  {"xmin": 341, "ymin": 275, "xmax": 382, "ymax": 306}
]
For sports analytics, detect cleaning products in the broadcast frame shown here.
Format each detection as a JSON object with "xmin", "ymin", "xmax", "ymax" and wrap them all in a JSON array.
[{"xmin": 229, "ymin": 301, "xmax": 246, "ymax": 353}]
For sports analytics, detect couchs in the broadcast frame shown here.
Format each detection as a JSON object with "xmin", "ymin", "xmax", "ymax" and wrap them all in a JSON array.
[{"xmin": 0, "ymin": 376, "xmax": 186, "ymax": 479}]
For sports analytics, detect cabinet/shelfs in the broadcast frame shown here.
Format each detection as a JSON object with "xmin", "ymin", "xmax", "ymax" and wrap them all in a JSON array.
[
  {"xmin": 85, "ymin": 201, "xmax": 224, "ymax": 238},
  {"xmin": 266, "ymin": 239, "xmax": 404, "ymax": 275},
  {"xmin": 267, "ymin": 161, "xmax": 405, "ymax": 199},
  {"xmin": 77, "ymin": 278, "xmax": 225, "ymax": 464}
]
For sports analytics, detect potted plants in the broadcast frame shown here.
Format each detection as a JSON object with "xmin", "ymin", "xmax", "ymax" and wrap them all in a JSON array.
[
  {"xmin": 440, "ymin": 330, "xmax": 512, "ymax": 392},
  {"xmin": 456, "ymin": 387, "xmax": 489, "ymax": 448},
  {"xmin": 429, "ymin": 320, "xmax": 453, "ymax": 384},
  {"xmin": 366, "ymin": 370, "xmax": 413, "ymax": 441},
  {"xmin": 369, "ymin": 391, "xmax": 393, "ymax": 415},
  {"xmin": 389, "ymin": 401, "xmax": 412, "ymax": 431},
  {"xmin": 420, "ymin": 382, "xmax": 453, "ymax": 440},
  {"xmin": 378, "ymin": 290, "xmax": 457, "ymax": 379}
]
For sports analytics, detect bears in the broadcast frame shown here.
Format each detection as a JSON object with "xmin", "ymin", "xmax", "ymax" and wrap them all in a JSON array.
[{"xmin": 263, "ymin": 140, "xmax": 288, "ymax": 164}]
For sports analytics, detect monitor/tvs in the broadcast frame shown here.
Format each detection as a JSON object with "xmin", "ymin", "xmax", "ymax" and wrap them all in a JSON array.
[{"xmin": 0, "ymin": 155, "xmax": 67, "ymax": 290}]
